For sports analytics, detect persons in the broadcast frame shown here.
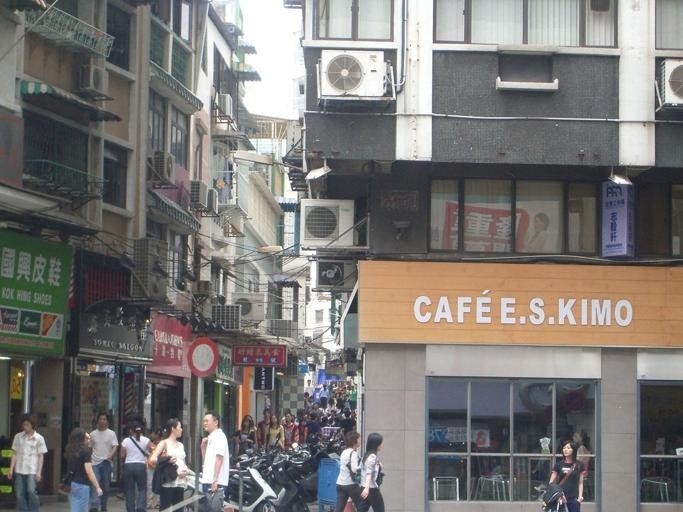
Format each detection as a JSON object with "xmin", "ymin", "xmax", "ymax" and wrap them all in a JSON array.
[
  {"xmin": 7, "ymin": 414, "xmax": 49, "ymax": 511},
  {"xmin": 146, "ymin": 425, "xmax": 165, "ymax": 510},
  {"xmin": 572, "ymin": 428, "xmax": 592, "ymax": 501},
  {"xmin": 522, "ymin": 212, "xmax": 550, "ymax": 255},
  {"xmin": 147, "ymin": 418, "xmax": 189, "ymax": 512},
  {"xmin": 548, "ymin": 440, "xmax": 586, "ymax": 511},
  {"xmin": 119, "ymin": 420, "xmax": 156, "ymax": 511},
  {"xmin": 357, "ymin": 433, "xmax": 384, "ymax": 511},
  {"xmin": 200, "ymin": 412, "xmax": 229, "ymax": 512},
  {"xmin": 232, "ymin": 379, "xmax": 358, "ymax": 462},
  {"xmin": 64, "ymin": 426, "xmax": 103, "ymax": 512},
  {"xmin": 88, "ymin": 412, "xmax": 119, "ymax": 512},
  {"xmin": 333, "ymin": 430, "xmax": 363, "ymax": 512}
]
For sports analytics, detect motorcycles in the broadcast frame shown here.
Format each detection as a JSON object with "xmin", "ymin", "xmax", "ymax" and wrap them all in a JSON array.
[{"xmin": 175, "ymin": 427, "xmax": 345, "ymax": 512}]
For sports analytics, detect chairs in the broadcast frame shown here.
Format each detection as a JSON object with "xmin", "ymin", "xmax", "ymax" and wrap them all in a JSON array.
[
  {"xmin": 433, "ymin": 447, "xmax": 595, "ymax": 501},
  {"xmin": 640, "ymin": 454, "xmax": 683, "ymax": 502}
]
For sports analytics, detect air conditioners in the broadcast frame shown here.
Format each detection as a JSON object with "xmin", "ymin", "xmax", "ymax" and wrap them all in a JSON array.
[
  {"xmin": 219, "ymin": 94, "xmax": 233, "ymax": 119},
  {"xmin": 660, "ymin": 59, "xmax": 683, "ymax": 106},
  {"xmin": 204, "ymin": 188, "xmax": 218, "ymax": 215},
  {"xmin": 233, "ymin": 293, "xmax": 265, "ymax": 320},
  {"xmin": 80, "ymin": 65, "xmax": 110, "ymax": 94},
  {"xmin": 192, "ymin": 181, "xmax": 208, "ymax": 209},
  {"xmin": 299, "ymin": 198, "xmax": 355, "ymax": 248},
  {"xmin": 152, "ymin": 151, "xmax": 176, "ymax": 186},
  {"xmin": 317, "ymin": 259, "xmax": 356, "ymax": 291},
  {"xmin": 321, "ymin": 49, "xmax": 386, "ymax": 98}
]
